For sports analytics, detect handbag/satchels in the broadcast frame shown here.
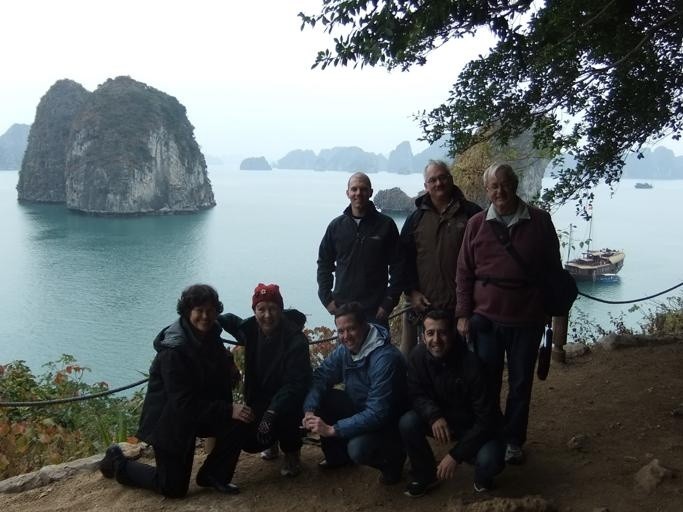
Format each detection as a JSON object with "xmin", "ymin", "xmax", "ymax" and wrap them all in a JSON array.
[{"xmin": 543, "ymin": 268, "xmax": 579, "ymax": 317}]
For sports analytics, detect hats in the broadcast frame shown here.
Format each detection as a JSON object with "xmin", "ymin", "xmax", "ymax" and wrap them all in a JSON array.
[{"xmin": 252, "ymin": 283, "xmax": 283, "ymax": 311}]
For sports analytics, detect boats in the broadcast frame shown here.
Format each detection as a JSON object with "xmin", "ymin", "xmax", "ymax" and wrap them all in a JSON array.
[
  {"xmin": 632, "ymin": 182, "xmax": 653, "ymax": 189},
  {"xmin": 564, "ymin": 212, "xmax": 625, "ymax": 282}
]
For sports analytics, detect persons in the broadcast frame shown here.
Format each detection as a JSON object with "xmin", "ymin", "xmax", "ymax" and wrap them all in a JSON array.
[
  {"xmin": 217, "ymin": 283, "xmax": 313, "ymax": 477},
  {"xmin": 99, "ymin": 285, "xmax": 257, "ymax": 499},
  {"xmin": 456, "ymin": 161, "xmax": 562, "ymax": 464},
  {"xmin": 318, "ymin": 176, "xmax": 400, "ymax": 333},
  {"xmin": 398, "ymin": 311, "xmax": 506, "ymax": 498},
  {"xmin": 401, "ymin": 163, "xmax": 484, "ymax": 351},
  {"xmin": 302, "ymin": 302, "xmax": 407, "ymax": 486}
]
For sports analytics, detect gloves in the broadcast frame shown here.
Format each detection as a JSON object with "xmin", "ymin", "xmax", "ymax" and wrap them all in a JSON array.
[{"xmin": 257, "ymin": 412, "xmax": 276, "ymax": 446}]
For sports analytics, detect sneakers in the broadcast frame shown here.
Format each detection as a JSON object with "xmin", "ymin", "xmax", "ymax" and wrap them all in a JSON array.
[
  {"xmin": 404, "ymin": 468, "xmax": 441, "ymax": 497},
  {"xmin": 196, "ymin": 473, "xmax": 239, "ymax": 493},
  {"xmin": 260, "ymin": 441, "xmax": 279, "ymax": 459},
  {"xmin": 504, "ymin": 442, "xmax": 525, "ymax": 464},
  {"xmin": 379, "ymin": 459, "xmax": 402, "ymax": 484},
  {"xmin": 473, "ymin": 480, "xmax": 487, "ymax": 492},
  {"xmin": 317, "ymin": 458, "xmax": 345, "ymax": 469},
  {"xmin": 280, "ymin": 448, "xmax": 301, "ymax": 477},
  {"xmin": 100, "ymin": 446, "xmax": 123, "ymax": 479}
]
